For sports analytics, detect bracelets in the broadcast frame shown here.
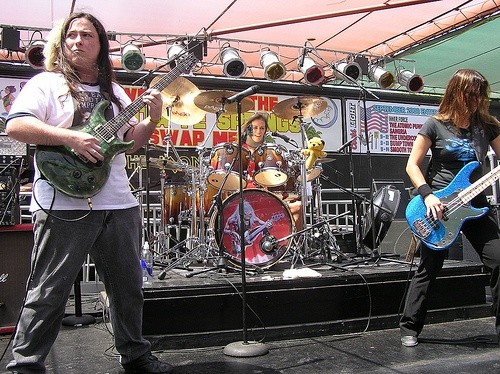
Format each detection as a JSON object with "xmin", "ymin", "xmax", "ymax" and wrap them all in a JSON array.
[
  {"xmin": 418, "ymin": 184, "xmax": 433, "ymax": 199},
  {"xmin": 149, "ymin": 119, "xmax": 160, "ymax": 123}
]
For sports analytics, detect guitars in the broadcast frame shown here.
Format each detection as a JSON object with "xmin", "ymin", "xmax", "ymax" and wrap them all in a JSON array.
[
  {"xmin": 404, "ymin": 160, "xmax": 500, "ymax": 252},
  {"xmin": 34, "ymin": 51, "xmax": 201, "ymax": 200}
]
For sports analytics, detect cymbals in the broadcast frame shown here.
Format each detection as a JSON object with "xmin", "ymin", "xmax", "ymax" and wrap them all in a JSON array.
[
  {"xmin": 285, "ymin": 158, "xmax": 337, "ymax": 168},
  {"xmin": 272, "ymin": 95, "xmax": 328, "ymax": 119},
  {"xmin": 193, "ymin": 89, "xmax": 255, "ymax": 115},
  {"xmin": 149, "ymin": 73, "xmax": 207, "ymax": 125}
]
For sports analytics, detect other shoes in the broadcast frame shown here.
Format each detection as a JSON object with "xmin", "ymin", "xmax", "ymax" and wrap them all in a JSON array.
[
  {"xmin": 122, "ymin": 351, "xmax": 173, "ymax": 374},
  {"xmin": 401, "ymin": 336, "xmax": 418, "ymax": 347}
]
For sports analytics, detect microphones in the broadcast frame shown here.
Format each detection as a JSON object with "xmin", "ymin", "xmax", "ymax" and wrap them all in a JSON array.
[
  {"xmin": 271, "ymin": 132, "xmax": 294, "ymax": 142},
  {"xmin": 337, "ymin": 136, "xmax": 357, "ymax": 152},
  {"xmin": 225, "ymin": 84, "xmax": 260, "ymax": 104},
  {"xmin": 204, "ymin": 30, "xmax": 207, "ymax": 56},
  {"xmin": 300, "ymin": 43, "xmax": 306, "ymax": 67}
]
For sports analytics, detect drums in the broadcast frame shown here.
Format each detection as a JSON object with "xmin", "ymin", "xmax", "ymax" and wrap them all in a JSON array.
[
  {"xmin": 251, "ymin": 142, "xmax": 291, "ymax": 188},
  {"xmin": 266, "ymin": 159, "xmax": 300, "ymax": 193},
  {"xmin": 162, "ymin": 181, "xmax": 202, "ymax": 268},
  {"xmin": 210, "ymin": 187, "xmax": 296, "ymax": 274},
  {"xmin": 200, "ymin": 179, "xmax": 230, "ymax": 241},
  {"xmin": 207, "ymin": 141, "xmax": 251, "ymax": 191},
  {"xmin": 290, "ymin": 147, "xmax": 324, "ymax": 183}
]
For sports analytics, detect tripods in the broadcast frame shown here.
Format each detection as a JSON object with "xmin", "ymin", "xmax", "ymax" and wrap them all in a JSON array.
[{"xmin": 153, "ymin": 50, "xmax": 416, "ymax": 280}]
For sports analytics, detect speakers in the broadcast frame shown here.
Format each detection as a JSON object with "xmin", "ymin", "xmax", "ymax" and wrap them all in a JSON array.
[{"xmin": 1, "ymin": 223, "xmax": 34, "ymax": 335}]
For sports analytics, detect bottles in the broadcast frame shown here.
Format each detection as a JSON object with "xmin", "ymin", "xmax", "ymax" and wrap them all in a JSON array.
[{"xmin": 141, "ymin": 241, "xmax": 153, "ymax": 285}]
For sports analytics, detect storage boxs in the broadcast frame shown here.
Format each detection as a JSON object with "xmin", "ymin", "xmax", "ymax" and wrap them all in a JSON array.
[{"xmin": 167, "ymin": 224, "xmax": 190, "ymax": 264}]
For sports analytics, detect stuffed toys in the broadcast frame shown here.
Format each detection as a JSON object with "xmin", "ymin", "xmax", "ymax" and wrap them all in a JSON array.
[{"xmin": 301, "ymin": 137, "xmax": 327, "ymax": 171}]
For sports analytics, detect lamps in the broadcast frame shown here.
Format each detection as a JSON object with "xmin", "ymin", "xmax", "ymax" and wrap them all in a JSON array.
[
  {"xmin": 366, "ymin": 65, "xmax": 395, "ymax": 90},
  {"xmin": 166, "ymin": 44, "xmax": 186, "ymax": 71},
  {"xmin": 25, "ymin": 37, "xmax": 49, "ymax": 70},
  {"xmin": 395, "ymin": 69, "xmax": 424, "ymax": 92},
  {"xmin": 298, "ymin": 56, "xmax": 325, "ymax": 85},
  {"xmin": 122, "ymin": 44, "xmax": 145, "ymax": 74},
  {"xmin": 332, "ymin": 59, "xmax": 362, "ymax": 84},
  {"xmin": 219, "ymin": 45, "xmax": 246, "ymax": 78},
  {"xmin": 259, "ymin": 50, "xmax": 287, "ymax": 83}
]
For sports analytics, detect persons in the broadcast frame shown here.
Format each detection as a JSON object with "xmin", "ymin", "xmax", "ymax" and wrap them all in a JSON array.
[
  {"xmin": 399, "ymin": 69, "xmax": 500, "ymax": 347},
  {"xmin": 2, "ymin": 12, "xmax": 173, "ymax": 374},
  {"xmin": 241, "ymin": 112, "xmax": 304, "ymax": 231}
]
